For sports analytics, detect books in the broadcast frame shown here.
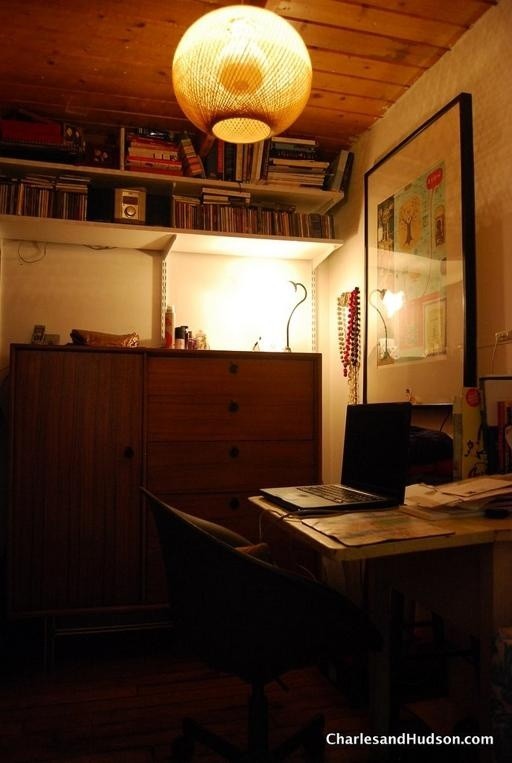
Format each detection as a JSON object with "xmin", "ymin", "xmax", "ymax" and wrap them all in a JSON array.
[{"xmin": 399, "ymin": 472, "xmax": 512, "ymax": 521}]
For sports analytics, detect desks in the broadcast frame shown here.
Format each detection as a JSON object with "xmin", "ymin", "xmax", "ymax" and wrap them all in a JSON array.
[{"xmin": 247, "ymin": 485, "xmax": 511, "ymax": 762}]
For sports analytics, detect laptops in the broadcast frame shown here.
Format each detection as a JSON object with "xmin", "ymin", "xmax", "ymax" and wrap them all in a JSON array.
[{"xmin": 259, "ymin": 403, "xmax": 413, "ymax": 517}]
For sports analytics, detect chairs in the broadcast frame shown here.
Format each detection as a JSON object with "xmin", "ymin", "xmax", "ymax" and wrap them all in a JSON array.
[{"xmin": 139, "ymin": 485, "xmax": 385, "ymax": 761}]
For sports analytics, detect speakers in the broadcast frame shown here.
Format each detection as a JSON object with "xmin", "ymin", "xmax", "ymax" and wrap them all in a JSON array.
[
  {"xmin": 87, "ymin": 188, "xmax": 114, "ymax": 221},
  {"xmin": 146, "ymin": 194, "xmax": 171, "ymax": 227}
]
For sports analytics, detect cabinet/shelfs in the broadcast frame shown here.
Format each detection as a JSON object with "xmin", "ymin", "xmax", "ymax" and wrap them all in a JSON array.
[
  {"xmin": 0, "ymin": 126, "xmax": 345, "ymax": 261},
  {"xmin": 3, "ymin": 343, "xmax": 322, "ymax": 675}
]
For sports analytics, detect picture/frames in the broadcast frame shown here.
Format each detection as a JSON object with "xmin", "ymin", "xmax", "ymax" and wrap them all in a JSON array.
[{"xmin": 361, "ymin": 92, "xmax": 477, "ymax": 412}]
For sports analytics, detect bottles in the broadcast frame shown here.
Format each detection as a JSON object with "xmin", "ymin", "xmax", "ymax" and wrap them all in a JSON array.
[{"xmin": 164, "ymin": 307, "xmax": 172, "ymax": 346}]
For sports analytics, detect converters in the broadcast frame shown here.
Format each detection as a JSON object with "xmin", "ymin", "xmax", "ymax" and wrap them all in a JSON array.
[{"xmin": 495, "ymin": 329, "xmax": 512, "ymax": 344}]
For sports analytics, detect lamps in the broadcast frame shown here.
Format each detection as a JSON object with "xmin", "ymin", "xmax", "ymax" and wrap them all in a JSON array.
[{"xmin": 169, "ymin": 1, "xmax": 313, "ymax": 145}]
